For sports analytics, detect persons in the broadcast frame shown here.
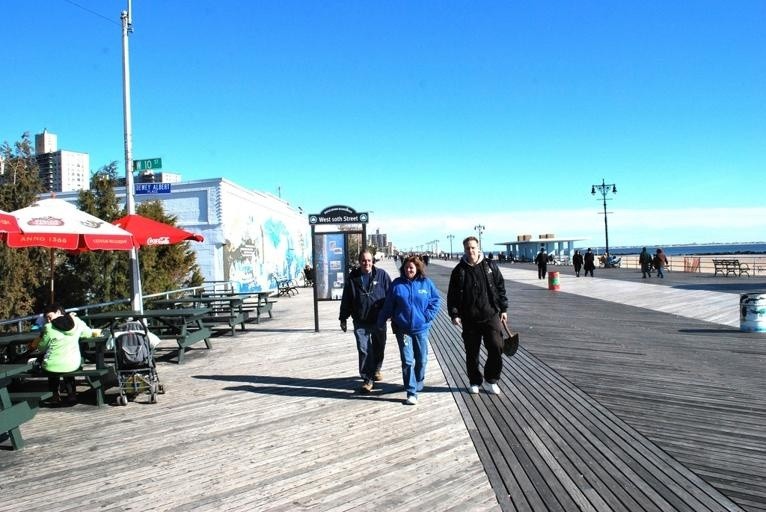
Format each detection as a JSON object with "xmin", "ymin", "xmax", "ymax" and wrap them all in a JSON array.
[
  {"xmin": 601, "ymin": 253, "xmax": 607, "ymax": 268},
  {"xmin": 639, "ymin": 246, "xmax": 652, "ymax": 278},
  {"xmin": 584, "ymin": 248, "xmax": 596, "ymax": 277},
  {"xmin": 447, "ymin": 236, "xmax": 508, "ymax": 394},
  {"xmin": 535, "ymin": 247, "xmax": 549, "ymax": 279},
  {"xmin": 376, "ymin": 255, "xmax": 442, "ymax": 405},
  {"xmin": 37, "ymin": 304, "xmax": 92, "ymax": 401},
  {"xmin": 655, "ymin": 248, "xmax": 668, "ymax": 278},
  {"xmin": 573, "ymin": 250, "xmax": 583, "ymax": 277},
  {"xmin": 379, "ymin": 254, "xmax": 384, "ymax": 263},
  {"xmin": 388, "ymin": 252, "xmax": 429, "ymax": 266},
  {"xmin": 339, "ymin": 248, "xmax": 392, "ymax": 391},
  {"xmin": 497, "ymin": 249, "xmax": 507, "ymax": 267},
  {"xmin": 510, "ymin": 251, "xmax": 513, "ymax": 261},
  {"xmin": 305, "ymin": 265, "xmax": 313, "ymax": 280},
  {"xmin": 488, "ymin": 252, "xmax": 493, "ymax": 260}
]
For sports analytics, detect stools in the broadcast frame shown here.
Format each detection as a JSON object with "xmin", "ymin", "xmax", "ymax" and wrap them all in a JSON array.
[
  {"xmin": 650, "ymin": 265, "xmax": 670, "ymax": 273},
  {"xmin": 712, "ymin": 259, "xmax": 750, "ymax": 277}
]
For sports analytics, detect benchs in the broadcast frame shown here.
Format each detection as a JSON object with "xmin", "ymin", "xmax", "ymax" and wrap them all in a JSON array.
[
  {"xmin": 8, "ymin": 391, "xmax": 56, "ymax": 409},
  {"xmin": 29, "ymin": 364, "xmax": 114, "ymax": 409}
]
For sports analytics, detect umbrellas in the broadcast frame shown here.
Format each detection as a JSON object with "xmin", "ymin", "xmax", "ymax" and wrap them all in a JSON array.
[
  {"xmin": 0, "ymin": 209, "xmax": 24, "ymax": 234},
  {"xmin": 92, "ymin": 214, "xmax": 204, "ymax": 311},
  {"xmin": 0, "ymin": 192, "xmax": 141, "ymax": 304}
]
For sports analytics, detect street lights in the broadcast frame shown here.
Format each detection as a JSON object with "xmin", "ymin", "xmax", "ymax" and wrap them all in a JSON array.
[
  {"xmin": 474, "ymin": 224, "xmax": 485, "ymax": 250},
  {"xmin": 400, "ymin": 239, "xmax": 440, "ymax": 259},
  {"xmin": 592, "ymin": 179, "xmax": 618, "ymax": 256},
  {"xmin": 474, "ymin": 225, "xmax": 485, "ymax": 253},
  {"xmin": 591, "ymin": 178, "xmax": 617, "ymax": 268},
  {"xmin": 447, "ymin": 234, "xmax": 455, "ymax": 259},
  {"xmin": 408, "ymin": 239, "xmax": 439, "ymax": 258},
  {"xmin": 447, "ymin": 234, "xmax": 456, "ymax": 260}
]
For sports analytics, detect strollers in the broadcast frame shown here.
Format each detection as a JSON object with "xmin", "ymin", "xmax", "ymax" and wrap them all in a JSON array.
[{"xmin": 110, "ymin": 317, "xmax": 166, "ymax": 405}]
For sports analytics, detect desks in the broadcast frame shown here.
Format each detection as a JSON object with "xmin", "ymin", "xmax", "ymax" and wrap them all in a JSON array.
[
  {"xmin": 0, "ymin": 362, "xmax": 36, "ymax": 453},
  {"xmin": 78, "ymin": 308, "xmax": 215, "ymax": 364},
  {"xmin": 150, "ymin": 296, "xmax": 252, "ymax": 336},
  {"xmin": 0, "ymin": 331, "xmax": 112, "ymax": 370},
  {"xmin": 190, "ymin": 292, "xmax": 277, "ymax": 325}
]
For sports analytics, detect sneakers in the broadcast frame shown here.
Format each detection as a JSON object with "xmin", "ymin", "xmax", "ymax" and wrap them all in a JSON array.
[
  {"xmin": 406, "ymin": 395, "xmax": 416, "ymax": 405},
  {"xmin": 360, "ymin": 382, "xmax": 373, "ymax": 394},
  {"xmin": 469, "ymin": 384, "xmax": 480, "ymax": 393},
  {"xmin": 374, "ymin": 371, "xmax": 382, "ymax": 381},
  {"xmin": 67, "ymin": 393, "xmax": 80, "ymax": 403},
  {"xmin": 52, "ymin": 391, "xmax": 61, "ymax": 403},
  {"xmin": 417, "ymin": 381, "xmax": 424, "ymax": 392},
  {"xmin": 482, "ymin": 381, "xmax": 500, "ymax": 394}
]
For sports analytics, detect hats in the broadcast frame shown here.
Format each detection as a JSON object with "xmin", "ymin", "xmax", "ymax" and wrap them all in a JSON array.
[
  {"xmin": 587, "ymin": 248, "xmax": 593, "ymax": 251},
  {"xmin": 643, "ymin": 247, "xmax": 646, "ymax": 251},
  {"xmin": 538, "ymin": 248, "xmax": 547, "ymax": 251}
]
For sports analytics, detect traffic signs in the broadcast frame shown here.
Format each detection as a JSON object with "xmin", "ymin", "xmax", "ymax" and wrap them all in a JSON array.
[
  {"xmin": 134, "ymin": 159, "xmax": 160, "ymax": 174},
  {"xmin": 134, "ymin": 183, "xmax": 171, "ymax": 193}
]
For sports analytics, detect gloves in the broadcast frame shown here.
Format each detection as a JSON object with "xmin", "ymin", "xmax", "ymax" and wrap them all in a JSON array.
[{"xmin": 340, "ymin": 321, "xmax": 347, "ymax": 332}]
